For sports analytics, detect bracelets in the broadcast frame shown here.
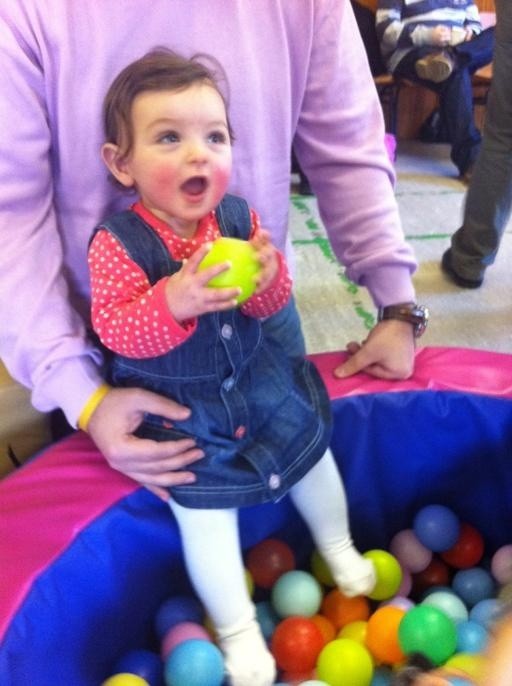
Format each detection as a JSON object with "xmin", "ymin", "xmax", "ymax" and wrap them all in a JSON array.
[{"xmin": 380, "ymin": 301, "xmax": 428, "ymax": 338}]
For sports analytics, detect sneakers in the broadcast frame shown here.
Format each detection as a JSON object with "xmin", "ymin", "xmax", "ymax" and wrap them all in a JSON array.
[
  {"xmin": 443, "ymin": 248, "xmax": 483, "ymax": 288},
  {"xmin": 415, "ymin": 53, "xmax": 454, "ymax": 83}
]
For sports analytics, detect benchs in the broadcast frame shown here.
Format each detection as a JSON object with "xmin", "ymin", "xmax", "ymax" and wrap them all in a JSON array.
[{"xmin": 374, "ymin": 63, "xmax": 492, "ymax": 161}]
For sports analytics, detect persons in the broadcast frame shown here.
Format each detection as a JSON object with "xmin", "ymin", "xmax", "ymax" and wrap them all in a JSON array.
[
  {"xmin": 83, "ymin": 46, "xmax": 379, "ymax": 683},
  {"xmin": 441, "ymin": 1, "xmax": 511, "ymax": 291},
  {"xmin": 1, "ymin": 0, "xmax": 426, "ymax": 504},
  {"xmin": 372, "ymin": 0, "xmax": 499, "ymax": 185}
]
